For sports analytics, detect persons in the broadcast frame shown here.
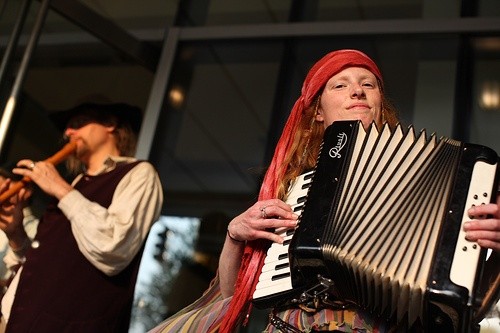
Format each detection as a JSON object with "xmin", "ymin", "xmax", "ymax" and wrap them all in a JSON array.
[
  {"xmin": 218, "ymin": 49, "xmax": 500, "ymax": 333},
  {"xmin": 0, "ymin": 93, "xmax": 163, "ymax": 333}
]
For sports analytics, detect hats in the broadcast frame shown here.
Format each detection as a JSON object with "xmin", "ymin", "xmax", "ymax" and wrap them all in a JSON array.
[
  {"xmin": 48, "ymin": 93, "xmax": 143, "ymax": 136},
  {"xmin": 198, "ymin": 211, "xmax": 230, "ymax": 242}
]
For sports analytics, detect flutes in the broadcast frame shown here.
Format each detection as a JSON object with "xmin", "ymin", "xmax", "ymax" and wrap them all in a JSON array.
[{"xmin": 0, "ymin": 142, "xmax": 77, "ymax": 206}]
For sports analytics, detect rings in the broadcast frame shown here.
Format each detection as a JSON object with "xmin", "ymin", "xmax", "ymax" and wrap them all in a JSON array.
[
  {"xmin": 31, "ymin": 163, "xmax": 36, "ymax": 170},
  {"xmin": 259, "ymin": 206, "xmax": 268, "ymax": 218}
]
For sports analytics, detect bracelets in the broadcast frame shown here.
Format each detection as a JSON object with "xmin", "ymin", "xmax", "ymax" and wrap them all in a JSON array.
[{"xmin": 227, "ymin": 229, "xmax": 244, "ymax": 242}]
